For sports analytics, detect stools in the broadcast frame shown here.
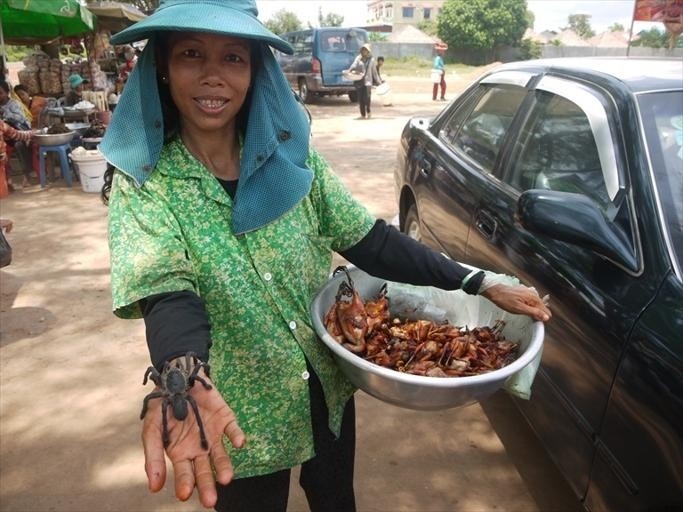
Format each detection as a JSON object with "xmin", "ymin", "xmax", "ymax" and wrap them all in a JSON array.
[{"xmin": 38, "ymin": 143, "xmax": 81, "ymax": 188}]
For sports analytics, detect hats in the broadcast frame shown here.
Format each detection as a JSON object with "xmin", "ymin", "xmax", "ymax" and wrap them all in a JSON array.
[
  {"xmin": 68, "ymin": 74, "xmax": 89, "ymax": 89},
  {"xmin": 359, "ymin": 43, "xmax": 371, "ymax": 53},
  {"xmin": 108, "ymin": 0, "xmax": 294, "ymax": 55}
]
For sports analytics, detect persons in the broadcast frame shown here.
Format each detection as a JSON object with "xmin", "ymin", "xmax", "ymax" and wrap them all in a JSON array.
[
  {"xmin": 0, "ymin": 108, "xmax": 38, "ymax": 199},
  {"xmin": 96, "ymin": 0, "xmax": 552, "ymax": 511},
  {"xmin": 371, "ymin": 56, "xmax": 392, "ymax": 106},
  {"xmin": 115, "ymin": 49, "xmax": 138, "ymax": 85},
  {"xmin": 66, "ymin": 74, "xmax": 91, "ymax": 107},
  {"xmin": 346, "ymin": 44, "xmax": 381, "ymax": 120},
  {"xmin": 0, "ymin": 80, "xmax": 33, "ymax": 193},
  {"xmin": 12, "ymin": 83, "xmax": 51, "ymax": 186},
  {"xmin": 431, "ymin": 49, "xmax": 446, "ymax": 102}
]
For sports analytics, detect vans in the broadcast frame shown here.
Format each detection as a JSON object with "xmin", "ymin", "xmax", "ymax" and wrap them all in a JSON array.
[{"xmin": 269, "ymin": 28, "xmax": 371, "ymax": 104}]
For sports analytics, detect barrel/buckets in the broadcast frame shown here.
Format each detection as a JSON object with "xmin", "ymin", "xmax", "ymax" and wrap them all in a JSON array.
[{"xmin": 71, "ymin": 150, "xmax": 109, "ymax": 193}]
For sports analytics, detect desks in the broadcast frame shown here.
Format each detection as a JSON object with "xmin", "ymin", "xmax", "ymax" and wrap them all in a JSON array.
[{"xmin": 48, "ymin": 107, "xmax": 98, "ymax": 123}]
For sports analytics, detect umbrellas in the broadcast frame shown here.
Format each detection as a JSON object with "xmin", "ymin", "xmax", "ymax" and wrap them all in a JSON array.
[
  {"xmin": 0, "ymin": 0, "xmax": 94, "ymax": 81},
  {"xmin": 85, "ymin": 1, "xmax": 150, "ymax": 36}
]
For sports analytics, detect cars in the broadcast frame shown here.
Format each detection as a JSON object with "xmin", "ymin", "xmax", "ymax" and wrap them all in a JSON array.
[{"xmin": 394, "ymin": 53, "xmax": 683, "ymax": 512}]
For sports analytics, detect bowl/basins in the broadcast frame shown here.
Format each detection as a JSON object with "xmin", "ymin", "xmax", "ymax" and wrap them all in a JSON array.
[
  {"xmin": 309, "ymin": 258, "xmax": 545, "ymax": 412},
  {"xmin": 31, "ymin": 122, "xmax": 91, "ymax": 146},
  {"xmin": 342, "ymin": 69, "xmax": 365, "ymax": 81}
]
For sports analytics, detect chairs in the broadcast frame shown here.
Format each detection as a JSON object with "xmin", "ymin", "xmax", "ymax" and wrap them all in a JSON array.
[
  {"xmin": 518, "ymin": 113, "xmax": 609, "ymax": 210},
  {"xmin": 463, "ymin": 85, "xmax": 524, "ymax": 172}
]
